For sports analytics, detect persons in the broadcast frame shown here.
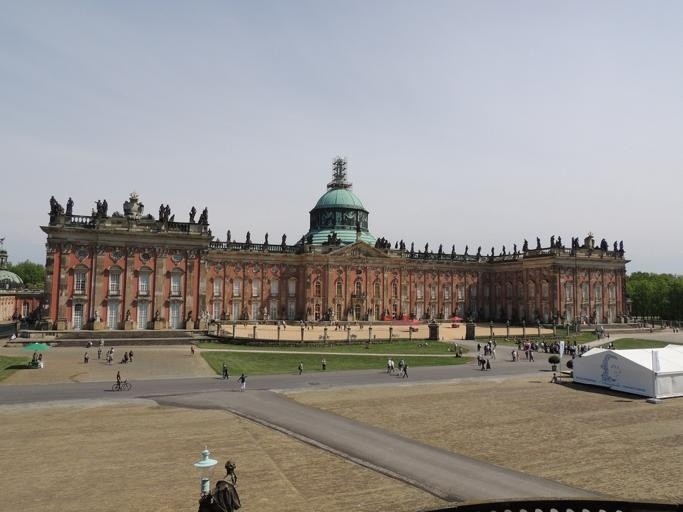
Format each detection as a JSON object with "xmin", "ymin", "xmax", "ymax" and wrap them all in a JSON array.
[
  {"xmin": 32, "ymin": 352, "xmax": 37, "ymax": 362},
  {"xmin": 386, "ymin": 357, "xmax": 409, "ymax": 378},
  {"xmin": 190, "ymin": 343, "xmax": 196, "ymax": 359},
  {"xmin": 221, "ymin": 362, "xmax": 229, "ymax": 379},
  {"xmin": 113, "ymin": 370, "xmax": 121, "ymax": 389},
  {"xmin": 237, "ymin": 371, "xmax": 247, "ymax": 391},
  {"xmin": 83, "ymin": 337, "xmax": 134, "ymax": 364},
  {"xmin": 207, "ymin": 460, "xmax": 241, "ymax": 511},
  {"xmin": 37, "ymin": 352, "xmax": 45, "ymax": 369},
  {"xmin": 448, "ymin": 338, "xmax": 591, "ymax": 373},
  {"xmin": 298, "ymin": 361, "xmax": 302, "ymax": 376},
  {"xmin": 321, "ymin": 356, "xmax": 327, "ymax": 370}
]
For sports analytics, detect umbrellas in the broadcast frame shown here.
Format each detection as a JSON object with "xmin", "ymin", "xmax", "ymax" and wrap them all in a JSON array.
[{"xmin": 20, "ymin": 343, "xmax": 49, "ymax": 369}]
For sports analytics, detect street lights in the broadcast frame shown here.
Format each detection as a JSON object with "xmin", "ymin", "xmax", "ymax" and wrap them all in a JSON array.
[{"xmin": 190, "ymin": 446, "xmax": 213, "ymax": 498}]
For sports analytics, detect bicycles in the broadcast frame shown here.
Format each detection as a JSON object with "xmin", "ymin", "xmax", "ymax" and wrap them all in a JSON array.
[{"xmin": 110, "ymin": 378, "xmax": 132, "ymax": 393}]
